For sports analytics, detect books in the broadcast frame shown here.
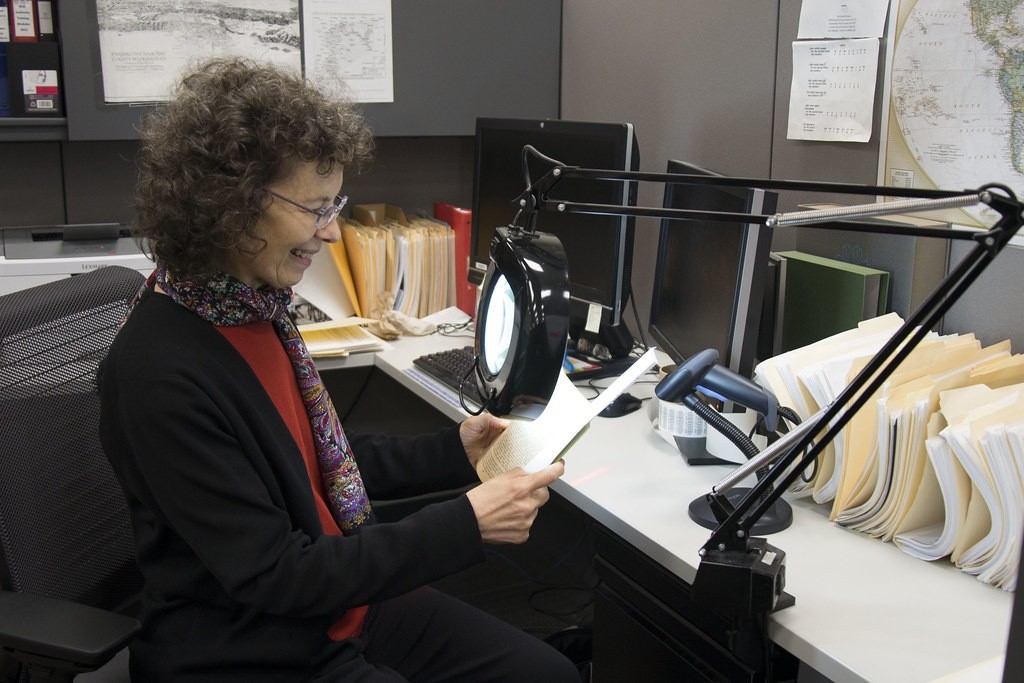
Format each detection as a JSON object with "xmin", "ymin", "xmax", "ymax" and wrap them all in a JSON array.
[
  {"xmin": 296, "ymin": 203, "xmax": 475, "ymax": 360},
  {"xmin": 752, "ymin": 310, "xmax": 1024, "ymax": 594}
]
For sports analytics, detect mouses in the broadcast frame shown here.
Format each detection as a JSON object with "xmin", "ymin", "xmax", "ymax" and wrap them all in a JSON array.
[{"xmin": 598, "ymin": 392, "xmax": 642, "ymax": 417}]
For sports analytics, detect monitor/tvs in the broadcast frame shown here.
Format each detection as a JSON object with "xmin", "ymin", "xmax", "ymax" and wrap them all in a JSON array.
[
  {"xmin": 468, "ymin": 115, "xmax": 640, "ymax": 377},
  {"xmin": 647, "ymin": 160, "xmax": 778, "ymax": 415}
]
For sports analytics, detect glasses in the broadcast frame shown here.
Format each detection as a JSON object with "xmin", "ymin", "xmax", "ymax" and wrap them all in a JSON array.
[{"xmin": 257, "ymin": 185, "xmax": 348, "ymax": 230}]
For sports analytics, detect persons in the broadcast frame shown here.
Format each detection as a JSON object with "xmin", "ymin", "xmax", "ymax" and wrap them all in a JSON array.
[{"xmin": 91, "ymin": 57, "xmax": 589, "ymax": 683}]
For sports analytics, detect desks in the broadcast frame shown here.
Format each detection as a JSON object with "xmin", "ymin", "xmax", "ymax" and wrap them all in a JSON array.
[
  {"xmin": 0, "ymin": 257, "xmax": 474, "ymax": 400},
  {"xmin": 376, "ymin": 332, "xmax": 1017, "ymax": 683}
]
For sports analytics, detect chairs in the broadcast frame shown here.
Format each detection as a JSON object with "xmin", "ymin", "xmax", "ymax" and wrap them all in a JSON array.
[{"xmin": 0, "ymin": 265, "xmax": 146, "ymax": 683}]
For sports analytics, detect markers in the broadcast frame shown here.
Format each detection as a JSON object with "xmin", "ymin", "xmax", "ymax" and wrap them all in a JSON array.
[{"xmin": 562, "ymin": 358, "xmax": 573, "ymax": 372}]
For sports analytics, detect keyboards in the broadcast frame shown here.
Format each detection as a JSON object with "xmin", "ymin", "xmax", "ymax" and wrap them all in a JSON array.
[{"xmin": 414, "ymin": 345, "xmax": 489, "ymax": 412}]
